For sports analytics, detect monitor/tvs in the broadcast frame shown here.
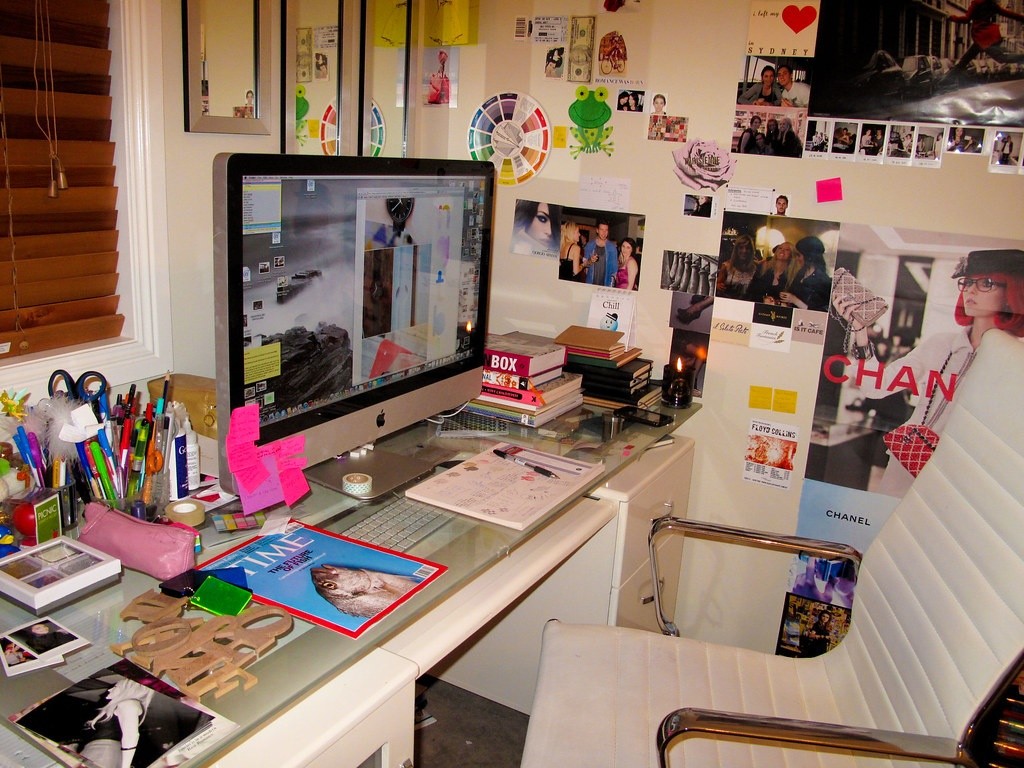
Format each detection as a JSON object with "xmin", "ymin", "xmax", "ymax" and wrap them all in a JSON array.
[{"xmin": 211, "ymin": 152, "xmax": 496, "ymax": 502}]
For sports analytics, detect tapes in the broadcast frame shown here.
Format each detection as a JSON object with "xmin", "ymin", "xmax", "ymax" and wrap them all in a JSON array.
[
  {"xmin": 342, "ymin": 474, "xmax": 372, "ymax": 494},
  {"xmin": 164, "ymin": 499, "xmax": 206, "ymax": 527}
]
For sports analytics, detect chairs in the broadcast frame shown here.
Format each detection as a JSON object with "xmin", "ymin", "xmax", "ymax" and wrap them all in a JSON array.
[{"xmin": 517, "ymin": 329, "xmax": 1024, "ymax": 768}]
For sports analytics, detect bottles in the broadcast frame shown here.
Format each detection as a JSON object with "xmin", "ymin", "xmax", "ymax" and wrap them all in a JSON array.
[
  {"xmin": 182, "ymin": 417, "xmax": 201, "ymax": 491},
  {"xmin": 168, "ymin": 419, "xmax": 188, "ymax": 501}
]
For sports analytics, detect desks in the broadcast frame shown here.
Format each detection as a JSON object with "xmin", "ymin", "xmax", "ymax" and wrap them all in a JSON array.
[{"xmin": 0, "ymin": 396, "xmax": 703, "ymax": 768}]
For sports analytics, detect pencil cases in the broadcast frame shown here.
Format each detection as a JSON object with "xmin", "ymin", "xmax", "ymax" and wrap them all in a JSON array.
[{"xmin": 77, "ymin": 501, "xmax": 202, "ymax": 582}]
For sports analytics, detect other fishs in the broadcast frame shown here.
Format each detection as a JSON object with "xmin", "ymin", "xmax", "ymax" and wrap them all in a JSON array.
[{"xmin": 310, "ymin": 564, "xmax": 419, "ymax": 620}]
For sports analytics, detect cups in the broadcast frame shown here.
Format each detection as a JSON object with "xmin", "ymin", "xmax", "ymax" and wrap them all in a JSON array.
[
  {"xmin": 661, "ymin": 364, "xmax": 696, "ymax": 409},
  {"xmin": 55, "ymin": 478, "xmax": 77, "ymax": 529},
  {"xmin": 602, "ymin": 410, "xmax": 625, "ymax": 444}
]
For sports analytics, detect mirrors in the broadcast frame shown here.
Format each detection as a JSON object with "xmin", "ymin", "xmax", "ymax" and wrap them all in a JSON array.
[
  {"xmin": 359, "ymin": 0, "xmax": 419, "ymax": 158},
  {"xmin": 182, "ymin": 0, "xmax": 273, "ymax": 135},
  {"xmin": 281, "ymin": 0, "xmax": 353, "ymax": 157}
]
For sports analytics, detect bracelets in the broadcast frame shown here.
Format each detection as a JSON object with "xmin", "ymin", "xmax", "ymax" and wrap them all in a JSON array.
[
  {"xmin": 120, "ymin": 746, "xmax": 137, "ymax": 751},
  {"xmin": 852, "ymin": 340, "xmax": 875, "ymax": 360}
]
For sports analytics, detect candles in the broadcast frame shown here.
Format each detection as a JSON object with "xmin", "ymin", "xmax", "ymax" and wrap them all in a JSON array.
[{"xmin": 661, "ymin": 358, "xmax": 697, "ymax": 408}]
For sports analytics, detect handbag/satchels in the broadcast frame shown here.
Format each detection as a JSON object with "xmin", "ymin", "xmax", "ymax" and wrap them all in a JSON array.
[
  {"xmin": 882, "ymin": 424, "xmax": 940, "ymax": 479},
  {"xmin": 829, "ymin": 267, "xmax": 889, "ymax": 327}
]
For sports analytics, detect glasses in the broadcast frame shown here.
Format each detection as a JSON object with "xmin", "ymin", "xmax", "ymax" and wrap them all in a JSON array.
[{"xmin": 958, "ymin": 278, "xmax": 1007, "ymax": 292}]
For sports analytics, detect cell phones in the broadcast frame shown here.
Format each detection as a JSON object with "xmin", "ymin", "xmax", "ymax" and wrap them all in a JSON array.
[{"xmin": 613, "ymin": 405, "xmax": 674, "ymax": 428}]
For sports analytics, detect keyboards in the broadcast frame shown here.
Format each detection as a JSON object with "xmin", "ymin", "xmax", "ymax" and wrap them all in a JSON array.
[{"xmin": 339, "ymin": 496, "xmax": 458, "ymax": 553}]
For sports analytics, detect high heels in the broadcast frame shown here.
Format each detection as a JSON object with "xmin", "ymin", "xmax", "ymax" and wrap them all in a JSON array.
[{"xmin": 677, "ymin": 308, "xmax": 701, "ymax": 324}]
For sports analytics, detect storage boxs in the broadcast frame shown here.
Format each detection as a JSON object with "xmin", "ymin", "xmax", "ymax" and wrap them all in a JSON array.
[
  {"xmin": 0, "ymin": 536, "xmax": 121, "ymax": 618},
  {"xmin": 7, "ymin": 486, "xmax": 63, "ymax": 546}
]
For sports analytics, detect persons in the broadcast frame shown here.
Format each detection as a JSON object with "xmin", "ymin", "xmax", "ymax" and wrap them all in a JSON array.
[
  {"xmin": 791, "ymin": 558, "xmax": 856, "ymax": 611},
  {"xmin": 809, "ymin": 610, "xmax": 835, "ymax": 642},
  {"xmin": 617, "ymin": 92, "xmax": 641, "ymax": 112},
  {"xmin": 717, "ymin": 235, "xmax": 832, "ymax": 313},
  {"xmin": 59, "ymin": 679, "xmax": 154, "ymax": 768},
  {"xmin": 691, "ymin": 196, "xmax": 711, "ymax": 218},
  {"xmin": 832, "ymin": 249, "xmax": 1024, "ymax": 499},
  {"xmin": 811, "ymin": 128, "xmax": 943, "ymax": 161},
  {"xmin": 738, "ymin": 65, "xmax": 810, "ymax": 108},
  {"xmin": 1000, "ymin": 136, "xmax": 1013, "ymax": 164},
  {"xmin": 775, "ymin": 195, "xmax": 789, "ymax": 216},
  {"xmin": 514, "ymin": 199, "xmax": 638, "ymax": 291},
  {"xmin": 736, "ymin": 115, "xmax": 802, "ymax": 159},
  {"xmin": 938, "ymin": 0, "xmax": 1024, "ymax": 85},
  {"xmin": 245, "ymin": 90, "xmax": 253, "ymax": 105},
  {"xmin": 4, "ymin": 640, "xmax": 26, "ymax": 664},
  {"xmin": 26, "ymin": 624, "xmax": 76, "ymax": 654},
  {"xmin": 949, "ymin": 128, "xmax": 972, "ymax": 152},
  {"xmin": 651, "ymin": 94, "xmax": 666, "ymax": 115}
]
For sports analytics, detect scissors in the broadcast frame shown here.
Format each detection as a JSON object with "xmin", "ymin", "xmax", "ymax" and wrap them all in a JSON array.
[{"xmin": 48, "ymin": 369, "xmax": 107, "ymax": 402}]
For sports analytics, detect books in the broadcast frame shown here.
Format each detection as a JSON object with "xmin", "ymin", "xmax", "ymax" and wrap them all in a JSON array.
[
  {"xmin": 406, "ymin": 441, "xmax": 605, "ymax": 531},
  {"xmin": 435, "ymin": 325, "xmax": 662, "ymax": 437},
  {"xmin": 644, "ymin": 434, "xmax": 675, "ymax": 451}
]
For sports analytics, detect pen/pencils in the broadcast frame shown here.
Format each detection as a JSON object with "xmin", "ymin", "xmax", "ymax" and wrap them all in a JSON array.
[
  {"xmin": 46, "ymin": 457, "xmax": 75, "ymax": 529},
  {"xmin": 72, "ymin": 383, "xmax": 175, "ymax": 511},
  {"xmin": 162, "ymin": 370, "xmax": 172, "ymax": 414},
  {"xmin": 492, "ymin": 449, "xmax": 560, "ymax": 479},
  {"xmin": 12, "ymin": 426, "xmax": 45, "ymax": 488}
]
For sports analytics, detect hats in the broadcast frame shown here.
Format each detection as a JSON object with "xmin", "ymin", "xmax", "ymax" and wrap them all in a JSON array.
[
  {"xmin": 951, "ymin": 249, "xmax": 1024, "ymax": 279},
  {"xmin": 795, "ymin": 236, "xmax": 825, "ymax": 257}
]
